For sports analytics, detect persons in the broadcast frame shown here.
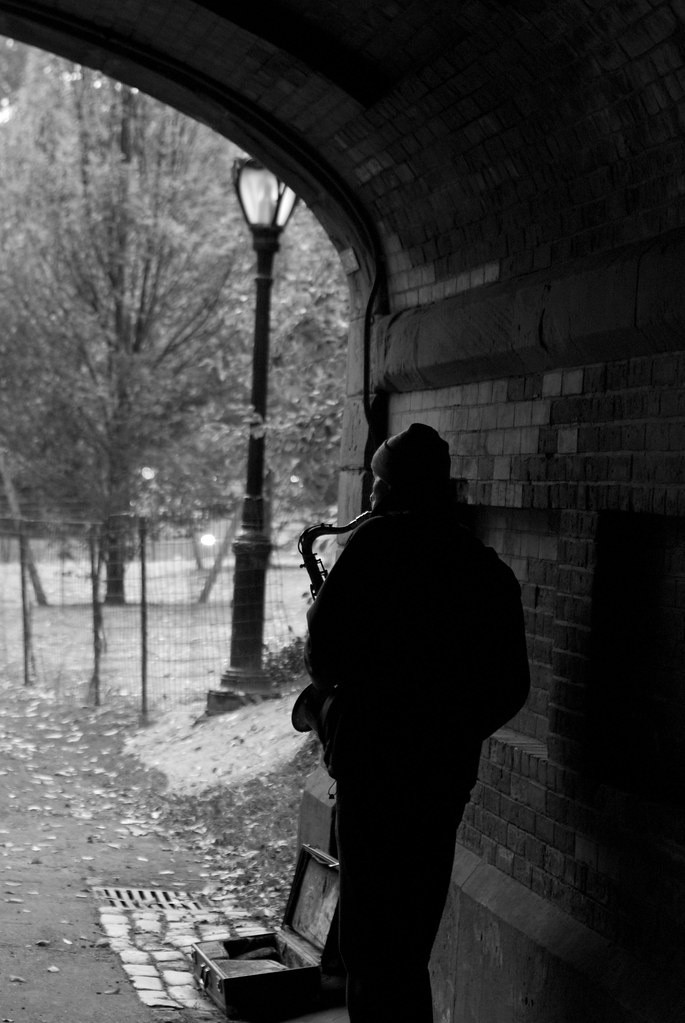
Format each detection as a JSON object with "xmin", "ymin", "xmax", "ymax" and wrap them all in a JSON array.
[
  {"xmin": 57, "ymin": 536, "xmax": 73, "ymax": 559},
  {"xmin": 291, "ymin": 419, "xmax": 531, "ymax": 1023}
]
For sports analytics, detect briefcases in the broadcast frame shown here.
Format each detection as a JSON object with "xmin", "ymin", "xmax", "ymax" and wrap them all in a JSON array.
[{"xmin": 190, "ymin": 844, "xmax": 343, "ymax": 1018}]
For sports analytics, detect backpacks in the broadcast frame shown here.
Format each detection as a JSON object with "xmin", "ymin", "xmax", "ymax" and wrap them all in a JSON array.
[{"xmin": 382, "ymin": 508, "xmax": 531, "ymax": 742}]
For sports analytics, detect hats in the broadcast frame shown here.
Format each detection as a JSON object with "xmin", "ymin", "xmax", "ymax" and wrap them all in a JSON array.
[{"xmin": 370, "ymin": 424, "xmax": 451, "ymax": 503}]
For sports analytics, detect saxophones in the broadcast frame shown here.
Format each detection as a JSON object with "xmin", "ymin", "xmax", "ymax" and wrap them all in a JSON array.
[{"xmin": 292, "ymin": 509, "xmax": 374, "ymax": 750}]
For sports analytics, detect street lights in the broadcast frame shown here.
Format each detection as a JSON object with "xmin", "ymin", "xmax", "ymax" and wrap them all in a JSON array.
[{"xmin": 206, "ymin": 154, "xmax": 300, "ymax": 719}]
